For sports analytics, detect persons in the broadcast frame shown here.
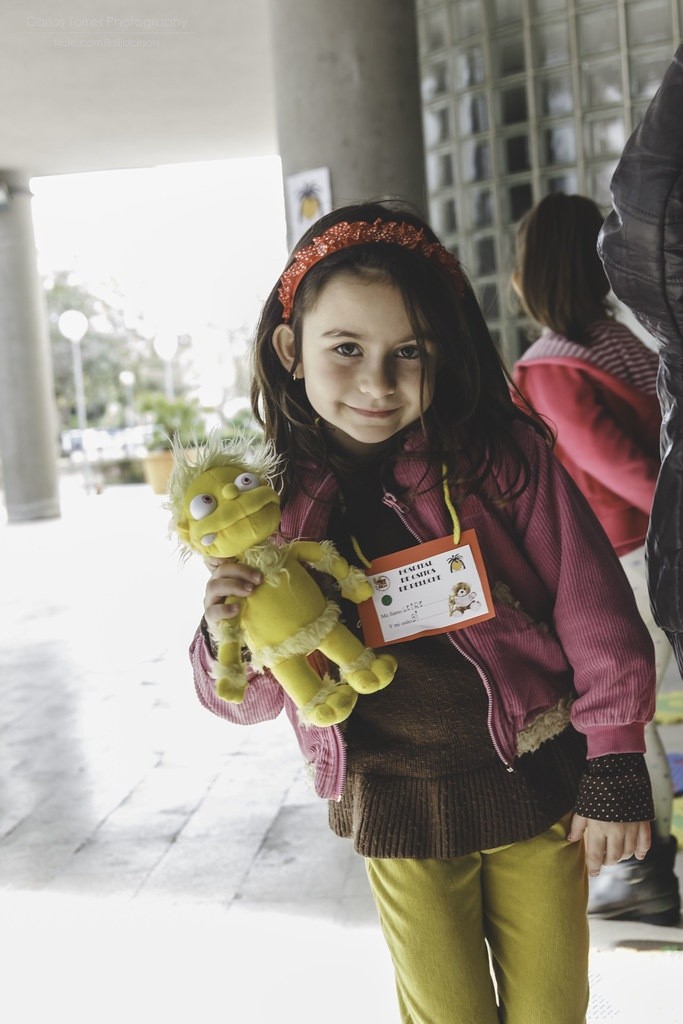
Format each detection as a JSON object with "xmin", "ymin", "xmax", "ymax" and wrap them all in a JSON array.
[
  {"xmin": 509, "ymin": 40, "xmax": 683, "ymax": 919},
  {"xmin": 190, "ymin": 201, "xmax": 656, "ymax": 1024}
]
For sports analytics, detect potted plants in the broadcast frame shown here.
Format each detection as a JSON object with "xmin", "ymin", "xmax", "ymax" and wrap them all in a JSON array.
[
  {"xmin": 134, "ymin": 389, "xmax": 179, "ymax": 494},
  {"xmin": 176, "ymin": 402, "xmax": 206, "ymax": 477}
]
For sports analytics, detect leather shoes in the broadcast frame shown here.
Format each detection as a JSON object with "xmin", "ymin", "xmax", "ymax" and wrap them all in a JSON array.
[{"xmin": 587, "ymin": 834, "xmax": 680, "ymax": 919}]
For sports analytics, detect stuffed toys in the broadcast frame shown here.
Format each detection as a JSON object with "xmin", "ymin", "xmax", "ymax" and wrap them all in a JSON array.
[{"xmin": 158, "ymin": 422, "xmax": 397, "ymax": 728}]
